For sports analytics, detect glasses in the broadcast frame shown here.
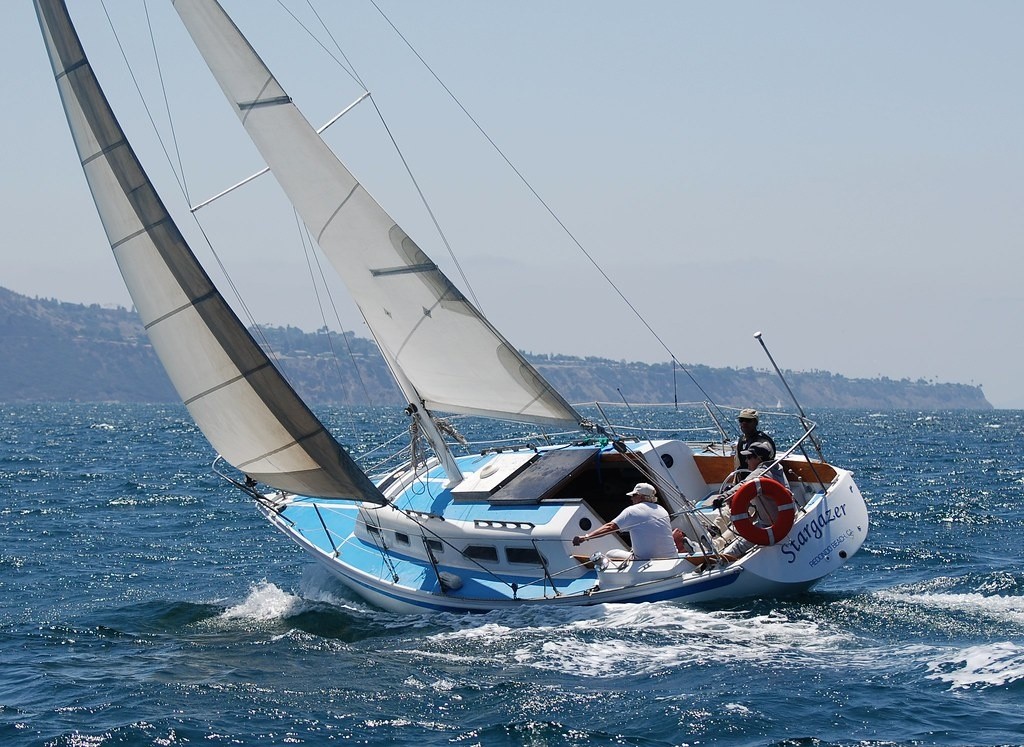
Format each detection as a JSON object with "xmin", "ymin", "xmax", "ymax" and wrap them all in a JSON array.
[
  {"xmin": 746, "ymin": 454, "xmax": 756, "ymax": 459},
  {"xmin": 739, "ymin": 417, "xmax": 753, "ymax": 423}
]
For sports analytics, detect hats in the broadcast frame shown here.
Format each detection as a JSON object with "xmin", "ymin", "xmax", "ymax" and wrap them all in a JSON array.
[
  {"xmin": 737, "ymin": 408, "xmax": 758, "ymax": 424},
  {"xmin": 625, "ymin": 483, "xmax": 656, "ymax": 497},
  {"xmin": 740, "ymin": 441, "xmax": 770, "ymax": 462}
]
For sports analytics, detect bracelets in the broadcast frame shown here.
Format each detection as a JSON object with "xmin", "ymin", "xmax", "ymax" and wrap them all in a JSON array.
[{"xmin": 585, "ymin": 533, "xmax": 589, "ymax": 541}]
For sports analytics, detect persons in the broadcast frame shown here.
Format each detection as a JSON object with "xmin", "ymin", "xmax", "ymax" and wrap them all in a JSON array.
[
  {"xmin": 683, "ymin": 409, "xmax": 776, "ymax": 556},
  {"xmin": 713, "ymin": 442, "xmax": 798, "ymax": 558},
  {"xmin": 573, "ymin": 482, "xmax": 679, "ymax": 558}
]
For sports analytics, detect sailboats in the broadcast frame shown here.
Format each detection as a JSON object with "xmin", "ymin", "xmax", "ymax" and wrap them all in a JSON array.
[{"xmin": 26, "ymin": 2, "xmax": 871, "ymax": 611}]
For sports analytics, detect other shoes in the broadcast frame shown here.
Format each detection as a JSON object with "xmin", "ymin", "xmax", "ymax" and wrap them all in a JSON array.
[
  {"xmin": 682, "ymin": 537, "xmax": 700, "ymax": 554},
  {"xmin": 700, "ymin": 534, "xmax": 717, "ymax": 555}
]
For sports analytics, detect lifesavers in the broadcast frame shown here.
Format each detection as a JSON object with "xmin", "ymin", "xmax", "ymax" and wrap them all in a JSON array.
[{"xmin": 730, "ymin": 478, "xmax": 795, "ymax": 546}]
